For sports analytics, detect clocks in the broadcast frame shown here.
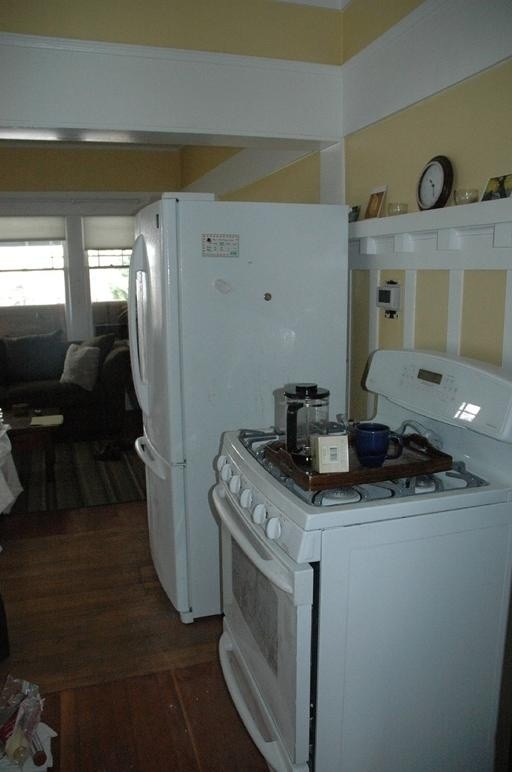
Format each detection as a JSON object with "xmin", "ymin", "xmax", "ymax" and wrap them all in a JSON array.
[{"xmin": 414, "ymin": 154, "xmax": 454, "ymax": 211}]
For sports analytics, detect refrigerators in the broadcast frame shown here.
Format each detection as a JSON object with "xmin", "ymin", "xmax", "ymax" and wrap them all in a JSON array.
[{"xmin": 126, "ymin": 189, "xmax": 350, "ymax": 627}]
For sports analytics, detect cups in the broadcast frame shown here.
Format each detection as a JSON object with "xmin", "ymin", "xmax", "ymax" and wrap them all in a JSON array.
[
  {"xmin": 387, "ymin": 200, "xmax": 408, "ymax": 216},
  {"xmin": 453, "ymin": 189, "xmax": 479, "ymax": 206},
  {"xmin": 353, "ymin": 423, "xmax": 403, "ymax": 468},
  {"xmin": 284, "ymin": 383, "xmax": 330, "ymax": 458}
]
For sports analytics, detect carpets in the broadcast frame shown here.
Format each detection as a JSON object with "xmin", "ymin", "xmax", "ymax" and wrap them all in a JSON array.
[
  {"xmin": 0, "ymin": 527, "xmax": 221, "ymax": 698},
  {"xmin": 12, "ymin": 436, "xmax": 146, "ymax": 514}
]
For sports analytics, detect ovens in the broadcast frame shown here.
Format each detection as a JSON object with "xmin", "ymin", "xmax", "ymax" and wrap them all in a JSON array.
[{"xmin": 206, "ymin": 478, "xmax": 318, "ymax": 770}]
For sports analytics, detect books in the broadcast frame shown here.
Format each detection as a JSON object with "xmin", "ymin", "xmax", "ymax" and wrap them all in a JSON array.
[{"xmin": 30, "ymin": 414, "xmax": 64, "ymax": 428}]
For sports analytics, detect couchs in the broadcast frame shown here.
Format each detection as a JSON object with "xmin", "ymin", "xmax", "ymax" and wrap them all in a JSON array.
[{"xmin": 1, "ymin": 338, "xmax": 139, "ymax": 439}]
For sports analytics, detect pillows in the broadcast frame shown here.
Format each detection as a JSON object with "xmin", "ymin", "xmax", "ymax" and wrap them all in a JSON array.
[
  {"xmin": 49, "ymin": 331, "xmax": 115, "ymax": 377},
  {"xmin": 2, "ymin": 330, "xmax": 65, "ymax": 383},
  {"xmin": 60, "ymin": 343, "xmax": 101, "ymax": 393}
]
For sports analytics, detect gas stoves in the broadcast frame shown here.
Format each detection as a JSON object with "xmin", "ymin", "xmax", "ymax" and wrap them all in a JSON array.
[{"xmin": 215, "ymin": 425, "xmax": 490, "ymax": 563}]
